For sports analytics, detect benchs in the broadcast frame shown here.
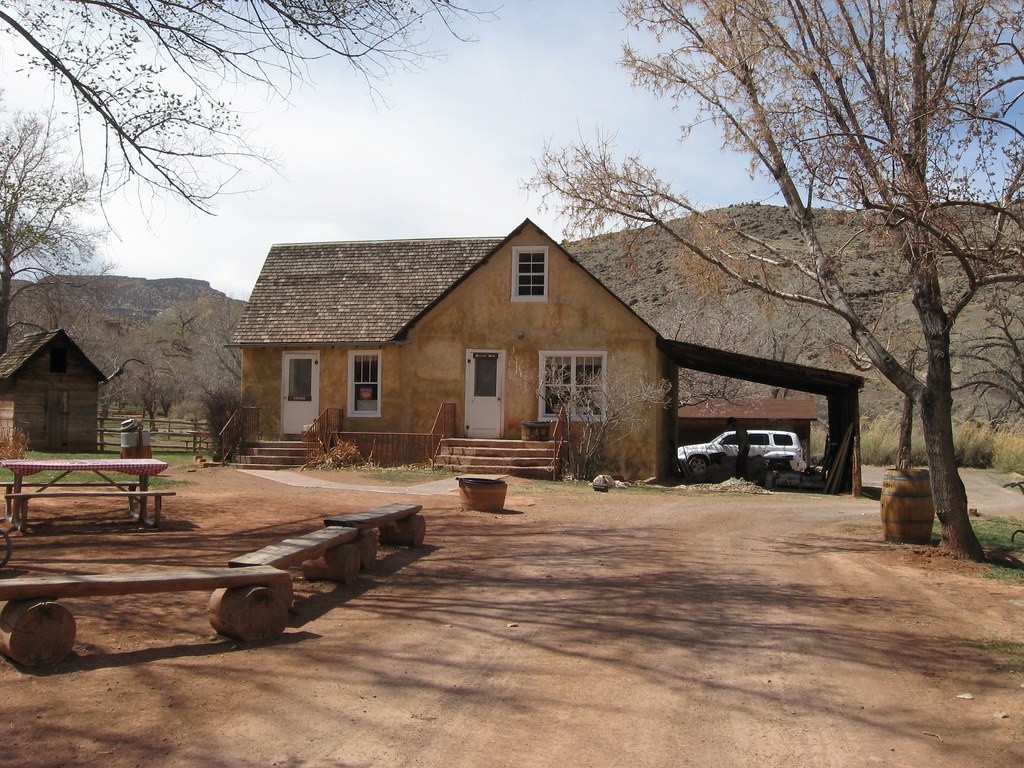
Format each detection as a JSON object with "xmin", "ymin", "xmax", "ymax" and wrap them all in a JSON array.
[
  {"xmin": 227, "ymin": 526, "xmax": 360, "ymax": 610},
  {"xmin": 322, "ymin": 502, "xmax": 427, "ymax": 572},
  {"xmin": 0, "ymin": 478, "xmax": 178, "ymax": 510},
  {"xmin": 0, "ymin": 563, "xmax": 292, "ymax": 668}
]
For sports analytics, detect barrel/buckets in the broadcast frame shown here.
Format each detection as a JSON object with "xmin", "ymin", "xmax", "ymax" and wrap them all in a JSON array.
[
  {"xmin": 880, "ymin": 468, "xmax": 934, "ymax": 544},
  {"xmin": 458, "ymin": 477, "xmax": 507, "ymax": 512},
  {"xmin": 521, "ymin": 420, "xmax": 549, "ymax": 441},
  {"xmin": 121, "ymin": 430, "xmax": 151, "ymax": 459}
]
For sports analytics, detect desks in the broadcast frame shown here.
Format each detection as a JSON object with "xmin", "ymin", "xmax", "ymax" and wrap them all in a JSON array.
[{"xmin": 0, "ymin": 458, "xmax": 178, "ymax": 533}]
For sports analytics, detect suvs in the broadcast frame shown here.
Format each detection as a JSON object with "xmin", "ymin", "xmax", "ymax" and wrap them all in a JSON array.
[{"xmin": 678, "ymin": 428, "xmax": 805, "ymax": 479}]
